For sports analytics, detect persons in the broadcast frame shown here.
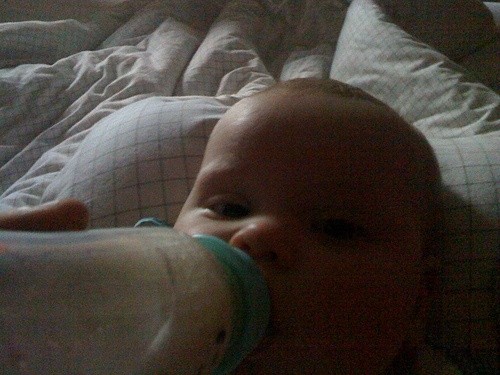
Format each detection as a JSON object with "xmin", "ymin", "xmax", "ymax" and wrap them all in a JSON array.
[
  {"xmin": 5, "ymin": 193, "xmax": 90, "ymax": 238},
  {"xmin": 162, "ymin": 76, "xmax": 462, "ymax": 375}
]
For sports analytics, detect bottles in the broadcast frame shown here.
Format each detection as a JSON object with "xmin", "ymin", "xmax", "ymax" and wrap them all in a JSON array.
[{"xmin": 0, "ymin": 218, "xmax": 277, "ymax": 374}]
